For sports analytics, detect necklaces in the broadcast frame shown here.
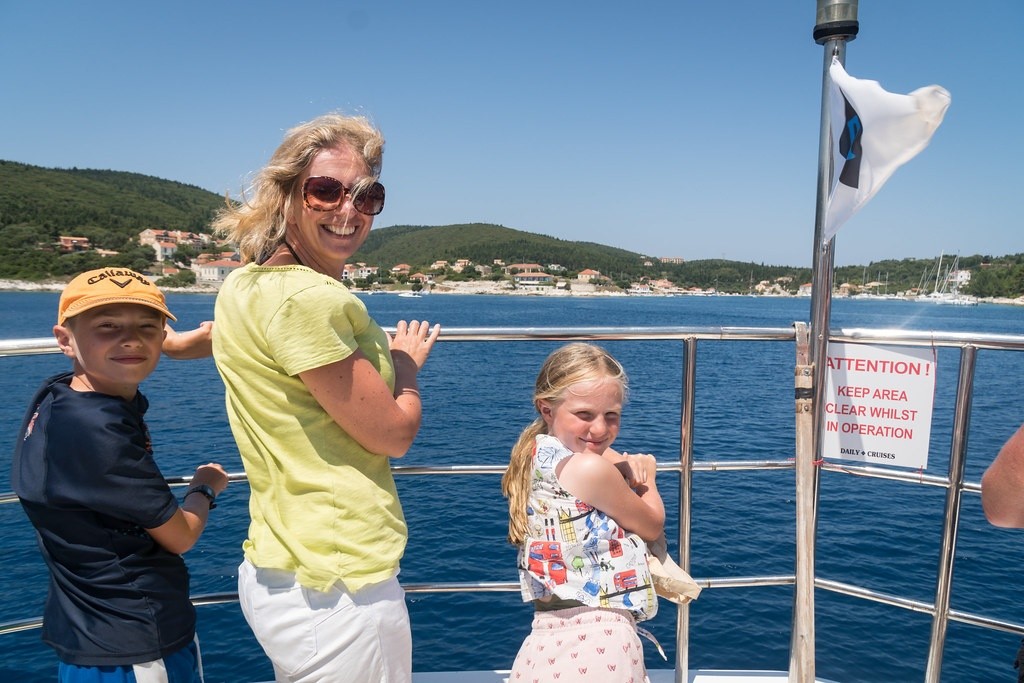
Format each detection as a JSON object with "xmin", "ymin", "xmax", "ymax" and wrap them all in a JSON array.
[{"xmin": 281, "ymin": 237, "xmax": 306, "ymax": 270}]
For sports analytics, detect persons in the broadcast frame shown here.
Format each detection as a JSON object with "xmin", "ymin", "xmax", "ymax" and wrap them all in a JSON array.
[
  {"xmin": 208, "ymin": 110, "xmax": 446, "ymax": 682},
  {"xmin": 11, "ymin": 265, "xmax": 230, "ymax": 682},
  {"xmin": 498, "ymin": 337, "xmax": 668, "ymax": 682},
  {"xmin": 977, "ymin": 413, "xmax": 1024, "ymax": 536}
]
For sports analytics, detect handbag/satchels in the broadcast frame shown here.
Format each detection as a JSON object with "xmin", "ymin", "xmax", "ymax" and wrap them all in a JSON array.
[{"xmin": 640, "ymin": 532, "xmax": 703, "ymax": 605}]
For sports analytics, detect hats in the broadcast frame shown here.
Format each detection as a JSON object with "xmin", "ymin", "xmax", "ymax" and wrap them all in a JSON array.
[{"xmin": 56, "ymin": 266, "xmax": 179, "ymax": 327}]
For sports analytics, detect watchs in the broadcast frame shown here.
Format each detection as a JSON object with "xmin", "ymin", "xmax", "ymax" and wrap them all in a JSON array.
[{"xmin": 181, "ymin": 485, "xmax": 220, "ymax": 511}]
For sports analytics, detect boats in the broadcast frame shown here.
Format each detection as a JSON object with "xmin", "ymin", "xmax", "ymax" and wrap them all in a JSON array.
[{"xmin": 860, "ymin": 252, "xmax": 980, "ymax": 308}]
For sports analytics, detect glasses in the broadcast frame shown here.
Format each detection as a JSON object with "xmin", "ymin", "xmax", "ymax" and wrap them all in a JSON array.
[{"xmin": 295, "ymin": 176, "xmax": 387, "ymax": 215}]
